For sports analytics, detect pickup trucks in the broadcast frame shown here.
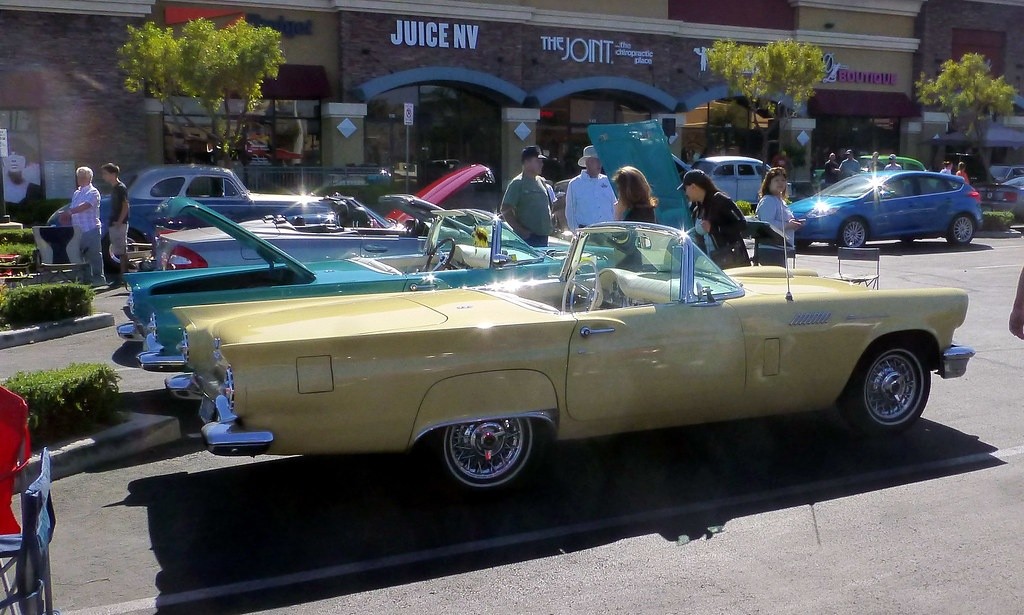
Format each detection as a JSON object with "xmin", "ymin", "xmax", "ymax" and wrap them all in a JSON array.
[{"xmin": 44, "ymin": 166, "xmax": 333, "ymax": 232}]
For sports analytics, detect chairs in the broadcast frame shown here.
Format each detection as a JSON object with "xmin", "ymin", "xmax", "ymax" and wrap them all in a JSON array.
[
  {"xmin": 32, "ymin": 226, "xmax": 88, "ymax": 284},
  {"xmin": 0, "ymin": 253, "xmax": 34, "ymax": 290},
  {"xmin": 824, "ymin": 247, "xmax": 880, "ymax": 290},
  {"xmin": 0, "ymin": 387, "xmax": 56, "ymax": 615}
]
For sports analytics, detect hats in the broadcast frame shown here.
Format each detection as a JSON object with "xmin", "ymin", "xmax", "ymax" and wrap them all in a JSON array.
[
  {"xmin": 677, "ymin": 170, "xmax": 705, "ymax": 190},
  {"xmin": 522, "ymin": 146, "xmax": 547, "ymax": 159},
  {"xmin": 577, "ymin": 146, "xmax": 599, "ymax": 167}
]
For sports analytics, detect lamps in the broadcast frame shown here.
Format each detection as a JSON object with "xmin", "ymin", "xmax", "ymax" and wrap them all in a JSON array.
[
  {"xmin": 523, "ymin": 96, "xmax": 540, "ymax": 108},
  {"xmin": 674, "ymin": 103, "xmax": 688, "ymax": 113}
]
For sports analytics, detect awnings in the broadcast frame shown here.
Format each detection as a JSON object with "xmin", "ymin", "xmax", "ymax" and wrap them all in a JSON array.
[
  {"xmin": 808, "ymin": 89, "xmax": 922, "ymax": 118},
  {"xmin": 172, "ymin": 64, "xmax": 333, "ymax": 101}
]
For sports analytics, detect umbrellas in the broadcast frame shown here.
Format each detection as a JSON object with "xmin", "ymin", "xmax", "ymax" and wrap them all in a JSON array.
[{"xmin": 915, "ymin": 121, "xmax": 1024, "ymax": 147}]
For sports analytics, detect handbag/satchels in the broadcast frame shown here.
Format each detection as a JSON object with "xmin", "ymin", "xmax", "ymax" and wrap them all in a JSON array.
[{"xmin": 710, "ymin": 248, "xmax": 735, "ymax": 267}]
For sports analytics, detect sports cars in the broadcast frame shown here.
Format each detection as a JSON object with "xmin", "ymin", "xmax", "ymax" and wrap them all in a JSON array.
[
  {"xmin": 164, "ymin": 222, "xmax": 981, "ymax": 504},
  {"xmin": 111, "ymin": 195, "xmax": 630, "ymax": 372},
  {"xmin": 137, "ymin": 191, "xmax": 454, "ymax": 275}
]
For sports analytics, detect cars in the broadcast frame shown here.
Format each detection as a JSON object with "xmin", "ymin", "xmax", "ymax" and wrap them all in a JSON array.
[
  {"xmin": 810, "ymin": 155, "xmax": 927, "ymax": 193},
  {"xmin": 425, "ymin": 158, "xmax": 496, "ymax": 188},
  {"xmin": 968, "ymin": 164, "xmax": 1024, "ymax": 220},
  {"xmin": 667, "ymin": 151, "xmax": 779, "ymax": 210},
  {"xmin": 786, "ymin": 168, "xmax": 983, "ymax": 249}
]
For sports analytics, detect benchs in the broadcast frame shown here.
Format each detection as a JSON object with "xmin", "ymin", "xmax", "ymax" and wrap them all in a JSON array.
[
  {"xmin": 351, "ymin": 257, "xmax": 403, "ymax": 275},
  {"xmin": 477, "ymin": 288, "xmax": 560, "ymax": 314}
]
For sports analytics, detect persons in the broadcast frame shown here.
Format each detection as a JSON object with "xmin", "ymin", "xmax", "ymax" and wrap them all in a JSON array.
[
  {"xmin": 1009, "ymin": 267, "xmax": 1024, "ymax": 340},
  {"xmin": 772, "ymin": 150, "xmax": 789, "ymax": 178},
  {"xmin": 939, "ymin": 160, "xmax": 953, "ymax": 174},
  {"xmin": 58, "ymin": 167, "xmax": 107, "ymax": 288},
  {"xmin": 884, "ymin": 154, "xmax": 902, "ymax": 170},
  {"xmin": 865, "ymin": 152, "xmax": 885, "ymax": 172},
  {"xmin": 101, "ymin": 163, "xmax": 130, "ymax": 288},
  {"xmin": 811, "ymin": 145, "xmax": 823, "ymax": 169},
  {"xmin": 892, "ymin": 181, "xmax": 908, "ymax": 198},
  {"xmin": 754, "ymin": 167, "xmax": 802, "ymax": 269},
  {"xmin": 956, "ymin": 162, "xmax": 968, "ymax": 184},
  {"xmin": 612, "ymin": 165, "xmax": 659, "ymax": 224},
  {"xmin": 839, "ymin": 149, "xmax": 861, "ymax": 180},
  {"xmin": 677, "ymin": 169, "xmax": 752, "ymax": 270},
  {"xmin": 825, "ymin": 152, "xmax": 839, "ymax": 187},
  {"xmin": 565, "ymin": 145, "xmax": 617, "ymax": 234},
  {"xmin": 500, "ymin": 146, "xmax": 554, "ymax": 247},
  {"xmin": 2, "ymin": 138, "xmax": 41, "ymax": 222}
]
